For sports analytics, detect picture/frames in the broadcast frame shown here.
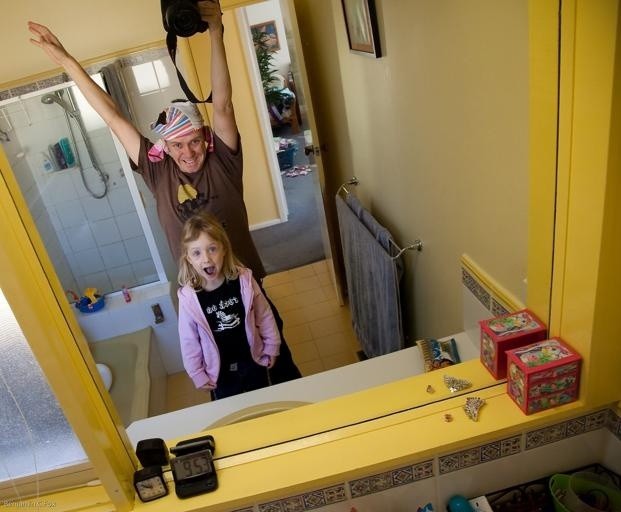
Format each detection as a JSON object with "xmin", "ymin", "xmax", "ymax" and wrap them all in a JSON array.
[
  {"xmin": 249, "ymin": 20, "xmax": 281, "ymax": 53},
  {"xmin": 340, "ymin": 0, "xmax": 383, "ymax": 59}
]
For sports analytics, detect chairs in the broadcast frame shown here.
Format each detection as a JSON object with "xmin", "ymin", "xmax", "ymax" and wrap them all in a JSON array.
[{"xmin": 267, "ymin": 71, "xmax": 303, "ymax": 134}]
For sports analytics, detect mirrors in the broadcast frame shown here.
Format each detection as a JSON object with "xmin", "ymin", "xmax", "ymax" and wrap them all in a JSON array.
[{"xmin": 2, "ymin": 1, "xmax": 560, "ymax": 474}]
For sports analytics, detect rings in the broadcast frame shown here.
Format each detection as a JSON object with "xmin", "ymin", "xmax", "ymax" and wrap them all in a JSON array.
[
  {"xmin": 425, "ymin": 383, "xmax": 434, "ymax": 394},
  {"xmin": 444, "ymin": 413, "xmax": 452, "ymax": 422}
]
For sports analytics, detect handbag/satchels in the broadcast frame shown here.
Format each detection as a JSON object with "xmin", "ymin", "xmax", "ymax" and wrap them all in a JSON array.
[{"xmin": 160, "ymin": 0, "xmax": 224, "ymax": 103}]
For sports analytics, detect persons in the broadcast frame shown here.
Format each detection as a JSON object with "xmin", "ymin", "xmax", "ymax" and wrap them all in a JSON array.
[
  {"xmin": 27, "ymin": 0, "xmax": 306, "ymax": 402},
  {"xmin": 176, "ymin": 210, "xmax": 284, "ymax": 403}
]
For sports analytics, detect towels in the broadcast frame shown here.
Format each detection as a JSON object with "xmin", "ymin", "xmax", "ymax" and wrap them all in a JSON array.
[
  {"xmin": 334, "ymin": 194, "xmax": 404, "ymax": 358},
  {"xmin": 345, "ymin": 192, "xmax": 403, "ymax": 283}
]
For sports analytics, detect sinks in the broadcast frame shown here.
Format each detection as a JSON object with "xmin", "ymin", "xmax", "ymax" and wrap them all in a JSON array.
[
  {"xmin": 201, "ymin": 399, "xmax": 313, "ymax": 430},
  {"xmin": 97, "ymin": 361, "xmax": 113, "ymax": 394}
]
